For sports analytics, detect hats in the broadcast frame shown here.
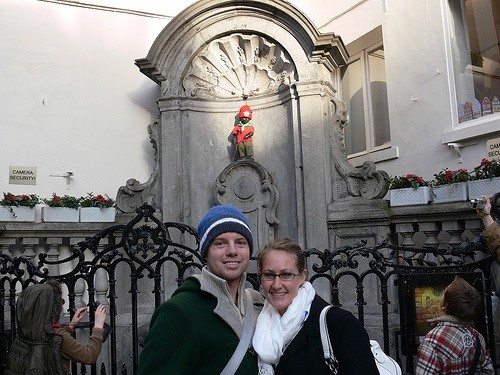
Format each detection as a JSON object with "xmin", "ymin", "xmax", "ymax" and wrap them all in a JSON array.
[
  {"xmin": 238, "ymin": 105, "xmax": 252, "ymax": 118},
  {"xmin": 198, "ymin": 205, "xmax": 253, "ymax": 263}
]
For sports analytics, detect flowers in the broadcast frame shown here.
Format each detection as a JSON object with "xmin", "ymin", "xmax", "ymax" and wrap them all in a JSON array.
[
  {"xmin": 40, "ymin": 192, "xmax": 81, "ymax": 210},
  {"xmin": 384, "ymin": 173, "xmax": 431, "ymax": 189},
  {"xmin": 0, "ymin": 191, "xmax": 39, "ymax": 218},
  {"xmin": 432, "ymin": 167, "xmax": 468, "ymax": 186},
  {"xmin": 468, "ymin": 158, "xmax": 500, "ymax": 182},
  {"xmin": 80, "ymin": 191, "xmax": 115, "ymax": 210}
]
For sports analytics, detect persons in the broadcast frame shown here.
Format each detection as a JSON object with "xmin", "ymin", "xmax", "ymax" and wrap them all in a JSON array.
[
  {"xmin": 415, "ymin": 275, "xmax": 495, "ymax": 375},
  {"xmin": 476, "ymin": 195, "xmax": 500, "ymax": 267},
  {"xmin": 251, "ymin": 236, "xmax": 380, "ymax": 375},
  {"xmin": 233, "ymin": 105, "xmax": 255, "ymax": 161},
  {"xmin": 138, "ymin": 204, "xmax": 265, "ymax": 375},
  {"xmin": 4, "ymin": 279, "xmax": 106, "ymax": 375}
]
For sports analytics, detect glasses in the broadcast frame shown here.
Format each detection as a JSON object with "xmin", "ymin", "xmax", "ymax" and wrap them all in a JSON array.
[{"xmin": 260, "ymin": 271, "xmax": 301, "ymax": 281}]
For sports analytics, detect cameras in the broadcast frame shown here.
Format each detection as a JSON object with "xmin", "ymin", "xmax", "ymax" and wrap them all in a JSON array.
[{"xmin": 471, "ymin": 198, "xmax": 486, "ymax": 210}]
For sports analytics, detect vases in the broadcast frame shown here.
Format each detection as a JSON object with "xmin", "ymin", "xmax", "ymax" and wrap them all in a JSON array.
[
  {"xmin": 41, "ymin": 207, "xmax": 80, "ymax": 222},
  {"xmin": 433, "ymin": 182, "xmax": 467, "ymax": 203},
  {"xmin": 390, "ymin": 187, "xmax": 432, "ymax": 206},
  {"xmin": 80, "ymin": 207, "xmax": 116, "ymax": 222},
  {"xmin": 469, "ymin": 177, "xmax": 500, "ymax": 201},
  {"xmin": 0, "ymin": 206, "xmax": 35, "ymax": 222}
]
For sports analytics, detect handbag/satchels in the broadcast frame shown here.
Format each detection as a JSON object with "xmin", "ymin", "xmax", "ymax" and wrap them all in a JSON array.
[{"xmin": 319, "ymin": 303, "xmax": 402, "ymax": 375}]
